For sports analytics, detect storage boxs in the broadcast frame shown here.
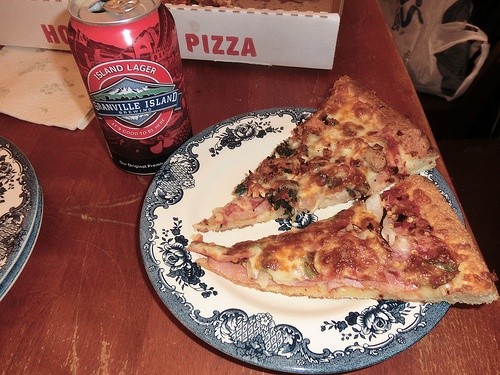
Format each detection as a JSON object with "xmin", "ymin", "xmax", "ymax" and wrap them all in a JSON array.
[{"xmin": 0, "ymin": 0, "xmax": 344, "ymax": 70}]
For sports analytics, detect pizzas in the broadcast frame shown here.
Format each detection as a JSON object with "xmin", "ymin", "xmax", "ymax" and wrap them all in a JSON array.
[
  {"xmin": 187, "ymin": 174, "xmax": 500, "ymax": 305},
  {"xmin": 191, "ymin": 76, "xmax": 441, "ymax": 233}
]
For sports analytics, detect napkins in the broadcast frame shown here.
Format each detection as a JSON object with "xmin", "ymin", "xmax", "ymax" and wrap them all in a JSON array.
[{"xmin": 0, "ymin": 46, "xmax": 96, "ymax": 131}]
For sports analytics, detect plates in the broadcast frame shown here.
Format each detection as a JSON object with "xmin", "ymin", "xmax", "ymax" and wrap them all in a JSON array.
[
  {"xmin": 0, "ymin": 136, "xmax": 44, "ymax": 301},
  {"xmin": 138, "ymin": 105, "xmax": 464, "ymax": 375}
]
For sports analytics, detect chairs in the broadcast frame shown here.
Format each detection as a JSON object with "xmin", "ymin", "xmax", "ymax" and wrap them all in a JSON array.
[{"xmin": 421, "ymin": 41, "xmax": 500, "ymax": 295}]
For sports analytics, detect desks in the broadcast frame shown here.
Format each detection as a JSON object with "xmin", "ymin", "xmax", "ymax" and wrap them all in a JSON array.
[{"xmin": 0, "ymin": 0, "xmax": 500, "ymax": 375}]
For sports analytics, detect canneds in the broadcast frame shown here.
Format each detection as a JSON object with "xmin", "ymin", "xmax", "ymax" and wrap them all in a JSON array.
[{"xmin": 66, "ymin": 0, "xmax": 193, "ymax": 176}]
